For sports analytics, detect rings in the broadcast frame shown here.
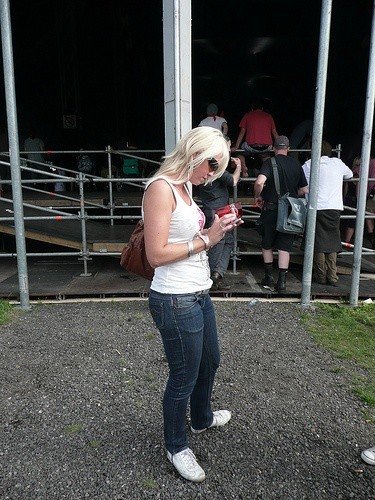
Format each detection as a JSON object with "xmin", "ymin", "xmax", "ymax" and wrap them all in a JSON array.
[{"xmin": 219, "ymin": 224, "xmax": 224, "ymax": 229}]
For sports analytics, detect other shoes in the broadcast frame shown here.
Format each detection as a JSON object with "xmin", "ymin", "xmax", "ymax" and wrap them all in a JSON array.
[{"xmin": 312, "ymin": 277, "xmax": 340, "ymax": 286}]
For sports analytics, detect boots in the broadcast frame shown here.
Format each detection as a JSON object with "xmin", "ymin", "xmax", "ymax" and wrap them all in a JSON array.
[
  {"xmin": 262, "ymin": 265, "xmax": 275, "ymax": 291},
  {"xmin": 275, "ymin": 270, "xmax": 288, "ymax": 291}
]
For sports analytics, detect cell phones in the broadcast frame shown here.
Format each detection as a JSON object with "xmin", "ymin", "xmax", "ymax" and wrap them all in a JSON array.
[{"xmin": 225, "ymin": 217, "xmax": 242, "ymax": 228}]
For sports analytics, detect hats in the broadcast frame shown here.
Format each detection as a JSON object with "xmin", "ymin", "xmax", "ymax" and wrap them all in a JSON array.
[{"xmin": 275, "ymin": 135, "xmax": 289, "ymax": 150}]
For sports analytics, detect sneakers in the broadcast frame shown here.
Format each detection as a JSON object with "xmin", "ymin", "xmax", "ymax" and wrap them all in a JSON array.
[
  {"xmin": 362, "ymin": 445, "xmax": 375, "ymax": 465},
  {"xmin": 190, "ymin": 410, "xmax": 231, "ymax": 433},
  {"xmin": 209, "ymin": 272, "xmax": 232, "ymax": 290},
  {"xmin": 168, "ymin": 447, "xmax": 205, "ymax": 482}
]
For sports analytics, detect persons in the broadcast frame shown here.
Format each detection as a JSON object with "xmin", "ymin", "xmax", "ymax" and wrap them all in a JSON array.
[
  {"xmin": 137, "ymin": 126, "xmax": 246, "ymax": 483},
  {"xmin": 230, "ymin": 101, "xmax": 280, "ymax": 181},
  {"xmin": 24, "ymin": 133, "xmax": 49, "ymax": 184},
  {"xmin": 353, "ymin": 156, "xmax": 375, "ymax": 242},
  {"xmin": 198, "ymin": 157, "xmax": 243, "ymax": 292},
  {"xmin": 196, "ymin": 104, "xmax": 228, "ymax": 136},
  {"xmin": 361, "ymin": 445, "xmax": 375, "ymax": 464},
  {"xmin": 298, "ymin": 143, "xmax": 354, "ymax": 284},
  {"xmin": 340, "ymin": 156, "xmax": 362, "ymax": 247},
  {"xmin": 253, "ymin": 134, "xmax": 310, "ymax": 293}
]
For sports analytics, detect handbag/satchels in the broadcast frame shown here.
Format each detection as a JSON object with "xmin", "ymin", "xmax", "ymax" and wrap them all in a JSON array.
[
  {"xmin": 277, "ymin": 192, "xmax": 306, "ymax": 235},
  {"xmin": 119, "ymin": 221, "xmax": 154, "ymax": 281}
]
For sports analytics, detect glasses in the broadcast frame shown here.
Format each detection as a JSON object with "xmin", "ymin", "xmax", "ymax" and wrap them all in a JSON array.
[{"xmin": 207, "ymin": 158, "xmax": 219, "ymax": 171}]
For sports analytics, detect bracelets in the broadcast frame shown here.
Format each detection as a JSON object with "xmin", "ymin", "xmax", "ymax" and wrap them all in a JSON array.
[
  {"xmin": 200, "ymin": 233, "xmax": 212, "ymax": 252},
  {"xmin": 187, "ymin": 240, "xmax": 195, "ymax": 257}
]
